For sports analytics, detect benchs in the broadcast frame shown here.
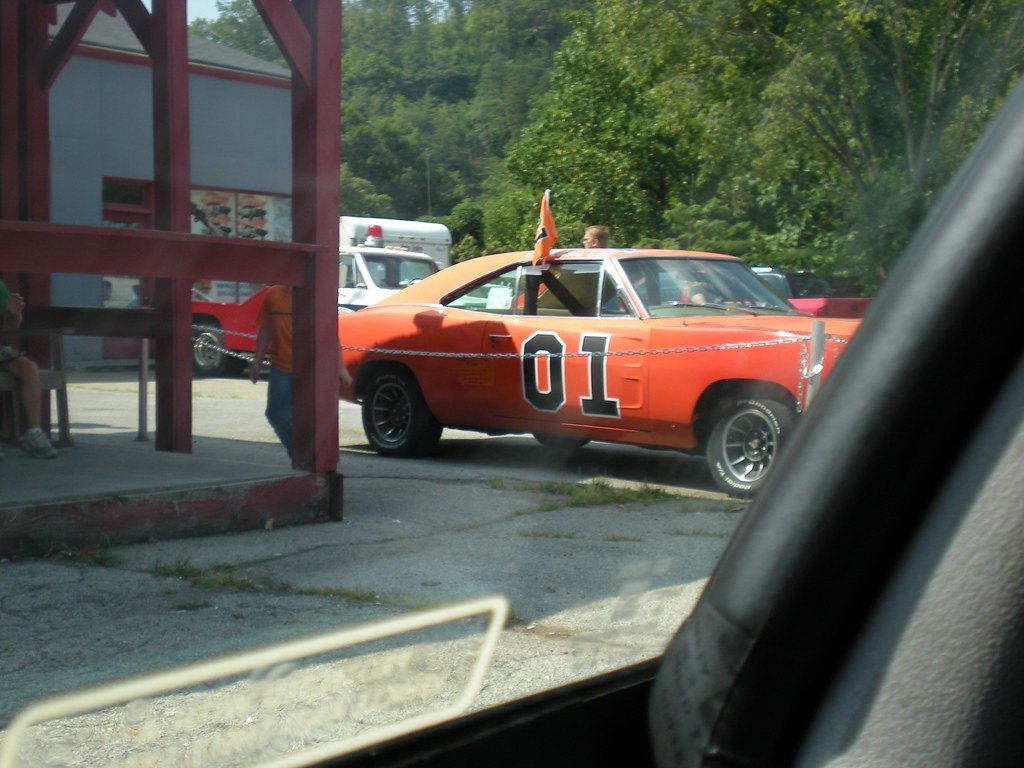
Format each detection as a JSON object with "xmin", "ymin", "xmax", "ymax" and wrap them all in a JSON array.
[{"xmin": 0, "ymin": 369, "xmax": 64, "ymax": 446}]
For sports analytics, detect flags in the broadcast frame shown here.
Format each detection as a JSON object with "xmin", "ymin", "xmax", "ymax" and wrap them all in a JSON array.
[{"xmin": 533, "ymin": 192, "xmax": 558, "ymax": 268}]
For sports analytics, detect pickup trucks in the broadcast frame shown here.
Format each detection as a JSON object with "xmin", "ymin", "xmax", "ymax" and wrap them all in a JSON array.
[{"xmin": 603, "ymin": 264, "xmax": 873, "ymax": 319}]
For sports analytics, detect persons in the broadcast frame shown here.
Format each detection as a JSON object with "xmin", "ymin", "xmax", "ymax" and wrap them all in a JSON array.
[
  {"xmin": 0, "ymin": 279, "xmax": 65, "ymax": 460},
  {"xmin": 250, "ymin": 285, "xmax": 353, "ymax": 460},
  {"xmin": 102, "ymin": 281, "xmax": 139, "ymax": 307},
  {"xmin": 583, "ymin": 226, "xmax": 618, "ymax": 311},
  {"xmin": 680, "ymin": 270, "xmax": 722, "ymax": 305}
]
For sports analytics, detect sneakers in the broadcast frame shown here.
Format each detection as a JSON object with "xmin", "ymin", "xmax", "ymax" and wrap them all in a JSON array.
[{"xmin": 22, "ymin": 430, "xmax": 58, "ymax": 459}]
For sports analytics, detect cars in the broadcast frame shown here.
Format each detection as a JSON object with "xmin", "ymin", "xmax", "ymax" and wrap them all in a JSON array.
[
  {"xmin": 338, "ymin": 247, "xmax": 874, "ymax": 499},
  {"xmin": 189, "ymin": 216, "xmax": 453, "ymax": 377}
]
